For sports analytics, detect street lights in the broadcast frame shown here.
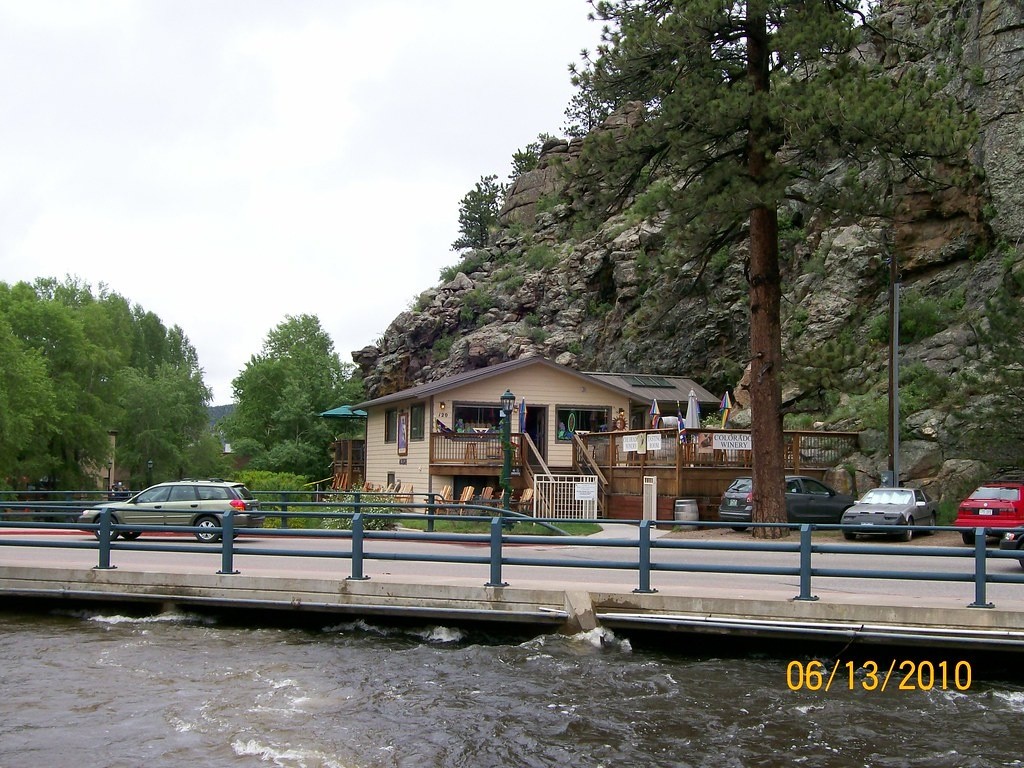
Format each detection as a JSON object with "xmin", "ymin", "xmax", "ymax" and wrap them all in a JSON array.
[
  {"xmin": 499, "ymin": 388, "xmax": 516, "ymax": 528},
  {"xmin": 147, "ymin": 459, "xmax": 153, "ymax": 487},
  {"xmin": 107, "ymin": 461, "xmax": 112, "ymax": 491}
]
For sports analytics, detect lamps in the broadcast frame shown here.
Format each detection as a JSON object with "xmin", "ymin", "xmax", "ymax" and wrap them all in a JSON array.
[
  {"xmin": 440, "ymin": 402, "xmax": 446, "ymax": 409},
  {"xmin": 619, "ymin": 407, "xmax": 625, "ymax": 416}
]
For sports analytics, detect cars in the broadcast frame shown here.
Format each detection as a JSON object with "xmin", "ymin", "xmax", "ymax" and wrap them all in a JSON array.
[{"xmin": 841, "ymin": 487, "xmax": 940, "ymax": 542}]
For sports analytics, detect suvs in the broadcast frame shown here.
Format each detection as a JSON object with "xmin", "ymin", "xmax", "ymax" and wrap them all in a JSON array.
[
  {"xmin": 717, "ymin": 475, "xmax": 856, "ymax": 531},
  {"xmin": 999, "ymin": 525, "xmax": 1024, "ymax": 568},
  {"xmin": 76, "ymin": 478, "xmax": 265, "ymax": 544},
  {"xmin": 952, "ymin": 479, "xmax": 1024, "ymax": 545}
]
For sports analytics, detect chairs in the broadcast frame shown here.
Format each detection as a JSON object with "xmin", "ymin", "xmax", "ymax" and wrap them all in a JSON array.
[{"xmin": 360, "ymin": 481, "xmax": 534, "ymax": 516}]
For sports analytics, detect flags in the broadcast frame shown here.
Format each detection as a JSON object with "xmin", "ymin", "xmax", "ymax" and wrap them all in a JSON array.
[
  {"xmin": 434, "ymin": 419, "xmax": 454, "ymax": 438},
  {"xmin": 677, "ymin": 407, "xmax": 686, "ymax": 444}
]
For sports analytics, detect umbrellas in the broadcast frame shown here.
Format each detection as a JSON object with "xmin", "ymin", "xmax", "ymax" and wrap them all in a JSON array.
[
  {"xmin": 519, "ymin": 397, "xmax": 527, "ymax": 433},
  {"xmin": 650, "ymin": 399, "xmax": 661, "ymax": 429},
  {"xmin": 720, "ymin": 391, "xmax": 734, "ymax": 428},
  {"xmin": 686, "ymin": 387, "xmax": 700, "ymax": 428}
]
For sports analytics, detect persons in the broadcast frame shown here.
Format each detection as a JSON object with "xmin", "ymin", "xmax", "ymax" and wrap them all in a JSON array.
[{"xmin": 108, "ymin": 480, "xmax": 130, "ymax": 501}]
